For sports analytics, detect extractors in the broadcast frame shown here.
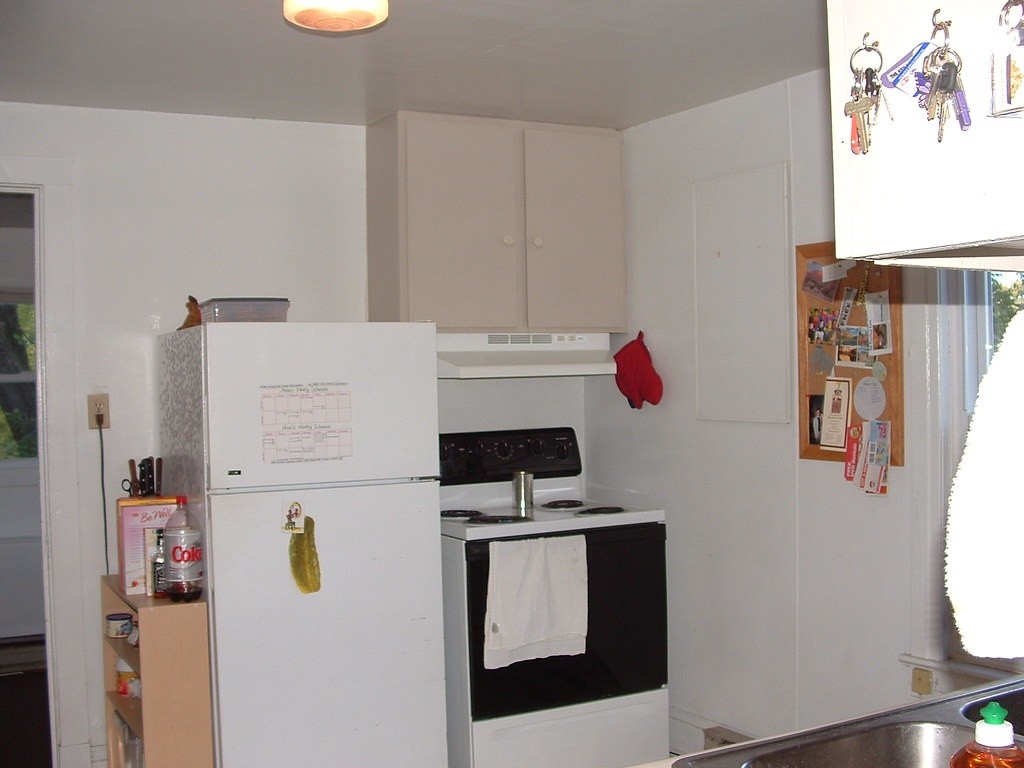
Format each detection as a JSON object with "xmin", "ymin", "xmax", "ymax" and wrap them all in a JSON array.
[{"xmin": 436, "ymin": 333, "xmax": 617, "ymax": 379}]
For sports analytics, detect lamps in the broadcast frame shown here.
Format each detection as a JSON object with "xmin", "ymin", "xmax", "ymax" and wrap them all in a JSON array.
[{"xmin": 281, "ymin": 0, "xmax": 390, "ymax": 33}]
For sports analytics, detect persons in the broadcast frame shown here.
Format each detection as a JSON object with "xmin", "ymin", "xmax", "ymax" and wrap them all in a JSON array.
[
  {"xmin": 810, "ymin": 308, "xmax": 838, "ymax": 344},
  {"xmin": 814, "ymin": 409, "xmax": 823, "ymax": 444},
  {"xmin": 873, "ymin": 324, "xmax": 888, "ymax": 350}
]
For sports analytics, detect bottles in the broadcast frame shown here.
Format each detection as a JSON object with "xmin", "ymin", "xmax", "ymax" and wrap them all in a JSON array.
[
  {"xmin": 164, "ymin": 496, "xmax": 204, "ymax": 602},
  {"xmin": 151, "ymin": 529, "xmax": 164, "ymax": 599}
]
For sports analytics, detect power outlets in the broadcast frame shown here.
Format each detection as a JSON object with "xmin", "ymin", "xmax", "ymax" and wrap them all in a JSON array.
[{"xmin": 88, "ymin": 394, "xmax": 109, "ymax": 430}]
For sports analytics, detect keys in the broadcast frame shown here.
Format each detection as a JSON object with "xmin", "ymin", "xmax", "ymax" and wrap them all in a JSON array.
[
  {"xmin": 923, "ymin": 47, "xmax": 971, "ymax": 142},
  {"xmin": 844, "ymin": 68, "xmax": 894, "ymax": 155}
]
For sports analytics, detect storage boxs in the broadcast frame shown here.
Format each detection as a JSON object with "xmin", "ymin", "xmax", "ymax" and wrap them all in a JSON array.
[{"xmin": 198, "ymin": 298, "xmax": 290, "ymax": 323}]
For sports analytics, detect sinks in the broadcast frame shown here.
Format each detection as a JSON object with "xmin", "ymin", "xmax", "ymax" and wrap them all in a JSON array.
[
  {"xmin": 961, "ymin": 686, "xmax": 1024, "ymax": 737},
  {"xmin": 741, "ymin": 719, "xmax": 974, "ymax": 768}
]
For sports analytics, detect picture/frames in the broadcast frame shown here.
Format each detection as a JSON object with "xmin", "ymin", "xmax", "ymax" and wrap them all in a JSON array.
[{"xmin": 794, "ymin": 239, "xmax": 905, "ymax": 469}]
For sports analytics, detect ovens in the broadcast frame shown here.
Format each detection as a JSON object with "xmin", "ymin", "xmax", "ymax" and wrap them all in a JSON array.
[{"xmin": 441, "ymin": 522, "xmax": 670, "ymax": 768}]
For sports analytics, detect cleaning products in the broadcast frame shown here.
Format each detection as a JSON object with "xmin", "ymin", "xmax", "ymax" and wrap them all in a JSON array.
[{"xmin": 950, "ymin": 700, "xmax": 1024, "ymax": 768}]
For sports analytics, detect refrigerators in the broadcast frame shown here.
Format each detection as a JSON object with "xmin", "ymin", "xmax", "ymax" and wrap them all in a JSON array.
[{"xmin": 157, "ymin": 322, "xmax": 449, "ymax": 768}]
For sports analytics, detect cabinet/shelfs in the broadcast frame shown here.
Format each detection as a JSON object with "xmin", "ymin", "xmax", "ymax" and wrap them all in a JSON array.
[
  {"xmin": 100, "ymin": 574, "xmax": 214, "ymax": 768},
  {"xmin": 826, "ymin": 0, "xmax": 1024, "ymax": 273},
  {"xmin": 363, "ymin": 108, "xmax": 627, "ymax": 335}
]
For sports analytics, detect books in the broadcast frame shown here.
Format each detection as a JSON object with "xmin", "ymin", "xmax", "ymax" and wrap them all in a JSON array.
[{"xmin": 116, "ymin": 497, "xmax": 188, "ymax": 597}]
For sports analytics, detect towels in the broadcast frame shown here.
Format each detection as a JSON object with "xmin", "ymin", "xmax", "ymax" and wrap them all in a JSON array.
[{"xmin": 483, "ymin": 533, "xmax": 588, "ymax": 669}]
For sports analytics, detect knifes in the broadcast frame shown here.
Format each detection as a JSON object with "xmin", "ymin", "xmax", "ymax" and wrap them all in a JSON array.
[{"xmin": 128, "ymin": 456, "xmax": 163, "ymax": 499}]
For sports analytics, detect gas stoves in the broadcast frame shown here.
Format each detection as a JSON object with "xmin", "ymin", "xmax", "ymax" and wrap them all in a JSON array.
[{"xmin": 439, "ymin": 427, "xmax": 665, "ymax": 541}]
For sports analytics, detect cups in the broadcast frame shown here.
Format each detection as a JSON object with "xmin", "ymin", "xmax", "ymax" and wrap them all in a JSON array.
[{"xmin": 512, "ymin": 471, "xmax": 534, "ymax": 509}]
